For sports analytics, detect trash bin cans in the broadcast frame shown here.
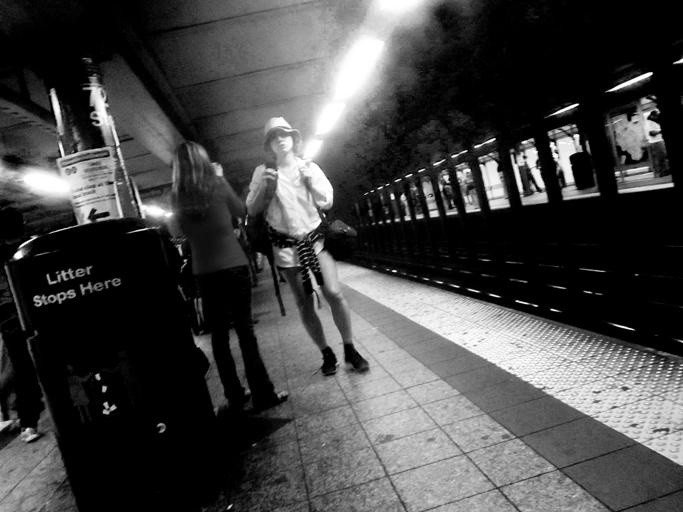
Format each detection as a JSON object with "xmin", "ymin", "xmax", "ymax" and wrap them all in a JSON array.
[{"xmin": 5, "ymin": 216, "xmax": 228, "ymax": 512}]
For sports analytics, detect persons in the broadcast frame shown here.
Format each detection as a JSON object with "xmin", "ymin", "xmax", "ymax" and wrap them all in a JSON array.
[
  {"xmin": 443, "ymin": 147, "xmax": 566, "ymax": 210},
  {"xmin": 644, "ymin": 110, "xmax": 670, "ymax": 177},
  {"xmin": 168, "ymin": 141, "xmax": 290, "ymax": 414},
  {"xmin": 245, "ymin": 115, "xmax": 370, "ymax": 377},
  {"xmin": 0, "ymin": 205, "xmax": 45, "ymax": 443}
]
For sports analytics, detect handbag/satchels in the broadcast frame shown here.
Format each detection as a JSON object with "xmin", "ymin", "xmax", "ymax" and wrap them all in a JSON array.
[
  {"xmin": 325, "ymin": 219, "xmax": 357, "ymax": 261},
  {"xmin": 179, "ymin": 257, "xmax": 200, "ymax": 301},
  {"xmin": 249, "ymin": 213, "xmax": 268, "ymax": 254}
]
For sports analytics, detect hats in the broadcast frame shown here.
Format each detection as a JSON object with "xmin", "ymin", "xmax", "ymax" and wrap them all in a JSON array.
[{"xmin": 262, "ymin": 116, "xmax": 301, "ymax": 150}]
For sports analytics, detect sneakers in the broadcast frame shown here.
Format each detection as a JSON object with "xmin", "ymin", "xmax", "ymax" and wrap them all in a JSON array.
[
  {"xmin": 255, "ymin": 390, "xmax": 288, "ymax": 410},
  {"xmin": 19, "ymin": 427, "xmax": 41, "ymax": 443},
  {"xmin": 321, "ymin": 356, "xmax": 339, "ymax": 376},
  {"xmin": 230, "ymin": 388, "xmax": 251, "ymax": 404},
  {"xmin": 345, "ymin": 349, "xmax": 369, "ymax": 371}
]
